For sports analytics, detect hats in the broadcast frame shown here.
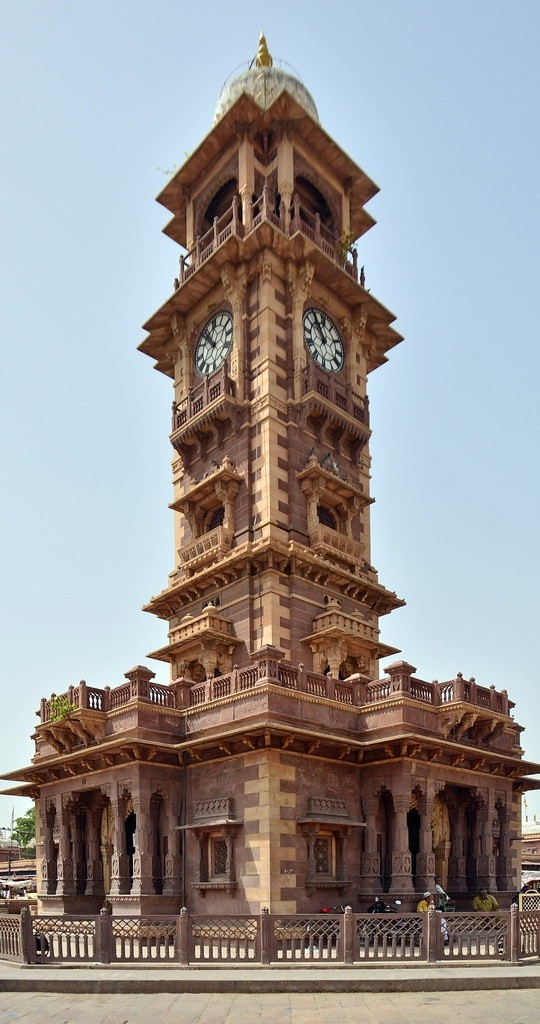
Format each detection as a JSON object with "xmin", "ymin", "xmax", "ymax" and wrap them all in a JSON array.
[{"xmin": 422, "ymin": 892, "xmax": 431, "ymax": 898}]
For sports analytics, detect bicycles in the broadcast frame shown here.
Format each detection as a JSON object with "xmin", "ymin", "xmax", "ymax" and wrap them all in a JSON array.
[{"xmin": 306, "ymin": 902, "xmax": 371, "ymax": 951}]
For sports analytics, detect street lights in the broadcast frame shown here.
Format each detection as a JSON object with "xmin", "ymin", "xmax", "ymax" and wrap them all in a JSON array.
[{"xmin": 2, "ymin": 826, "xmax": 13, "ymax": 881}]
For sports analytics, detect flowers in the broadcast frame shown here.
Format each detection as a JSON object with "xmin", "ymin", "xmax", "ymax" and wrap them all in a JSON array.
[{"xmin": 334, "ymin": 227, "xmax": 356, "ymax": 262}]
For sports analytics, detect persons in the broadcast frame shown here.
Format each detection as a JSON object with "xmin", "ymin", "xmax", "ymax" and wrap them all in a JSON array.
[
  {"xmin": 416, "ymin": 891, "xmax": 449, "ymax": 946},
  {"xmin": 473, "ymin": 889, "xmax": 504, "ymax": 930},
  {"xmin": 511, "ymin": 885, "xmax": 540, "ymax": 910}
]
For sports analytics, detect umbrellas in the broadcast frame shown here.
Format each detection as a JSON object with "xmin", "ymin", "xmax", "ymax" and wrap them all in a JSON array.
[{"xmin": 525, "ymin": 877, "xmax": 540, "ymax": 889}]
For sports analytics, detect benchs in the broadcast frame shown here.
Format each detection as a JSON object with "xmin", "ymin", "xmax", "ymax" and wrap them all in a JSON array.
[{"xmin": 438, "ymin": 894, "xmax": 456, "ymax": 912}]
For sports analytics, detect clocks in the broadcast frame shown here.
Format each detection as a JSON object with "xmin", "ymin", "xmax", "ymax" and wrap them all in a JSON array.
[
  {"xmin": 302, "ymin": 307, "xmax": 346, "ymax": 374},
  {"xmin": 193, "ymin": 309, "xmax": 234, "ymax": 379}
]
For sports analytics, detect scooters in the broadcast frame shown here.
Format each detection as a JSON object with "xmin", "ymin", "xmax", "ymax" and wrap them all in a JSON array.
[{"xmin": 370, "ymin": 897, "xmax": 450, "ymax": 950}]
